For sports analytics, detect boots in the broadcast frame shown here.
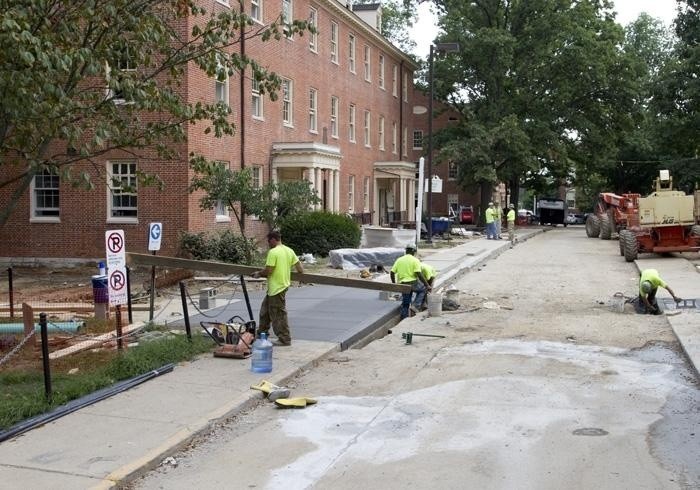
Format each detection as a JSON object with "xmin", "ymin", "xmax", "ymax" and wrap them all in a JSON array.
[{"xmin": 250, "ymin": 379, "xmax": 317, "ymax": 408}]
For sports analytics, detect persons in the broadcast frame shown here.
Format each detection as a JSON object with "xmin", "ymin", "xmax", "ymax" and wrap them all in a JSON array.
[
  {"xmin": 486, "ymin": 202, "xmax": 498, "ymax": 239},
  {"xmin": 506, "ymin": 203, "xmax": 516, "ymax": 241},
  {"xmin": 416, "ymin": 263, "xmax": 436, "ymax": 309},
  {"xmin": 390, "ymin": 243, "xmax": 432, "ymax": 319},
  {"xmin": 250, "ymin": 231, "xmax": 304, "ymax": 346},
  {"xmin": 493, "ymin": 200, "xmax": 506, "ymax": 239},
  {"xmin": 639, "ymin": 268, "xmax": 682, "ymax": 313}
]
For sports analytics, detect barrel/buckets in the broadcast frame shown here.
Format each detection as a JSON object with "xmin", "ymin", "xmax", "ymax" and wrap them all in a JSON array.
[
  {"xmin": 251, "ymin": 332, "xmax": 273, "ymax": 373},
  {"xmin": 427, "ymin": 294, "xmax": 442, "ymax": 316},
  {"xmin": 91, "ymin": 276, "xmax": 109, "ymax": 303}
]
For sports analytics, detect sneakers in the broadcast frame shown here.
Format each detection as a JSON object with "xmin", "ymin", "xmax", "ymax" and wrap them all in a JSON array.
[{"xmin": 270, "ymin": 339, "xmax": 291, "ymax": 346}]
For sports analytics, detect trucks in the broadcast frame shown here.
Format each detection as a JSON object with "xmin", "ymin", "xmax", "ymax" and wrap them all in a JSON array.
[
  {"xmin": 518, "ymin": 209, "xmax": 533, "ymax": 217},
  {"xmin": 539, "ymin": 199, "xmax": 568, "ymax": 227}
]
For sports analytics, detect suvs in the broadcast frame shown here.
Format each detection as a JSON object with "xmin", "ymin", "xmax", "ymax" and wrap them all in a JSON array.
[{"xmin": 567, "ymin": 214, "xmax": 584, "ymax": 225}]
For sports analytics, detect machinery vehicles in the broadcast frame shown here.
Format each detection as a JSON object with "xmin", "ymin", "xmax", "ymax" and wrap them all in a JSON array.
[{"xmin": 585, "ymin": 170, "xmax": 700, "ymax": 262}]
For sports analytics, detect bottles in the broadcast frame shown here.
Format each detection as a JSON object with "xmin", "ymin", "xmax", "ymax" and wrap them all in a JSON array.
[{"xmin": 99, "ymin": 262, "xmax": 106, "ymax": 275}]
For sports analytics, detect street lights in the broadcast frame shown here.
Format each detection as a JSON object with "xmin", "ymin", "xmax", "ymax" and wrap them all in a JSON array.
[{"xmin": 424, "ymin": 42, "xmax": 460, "ymax": 244}]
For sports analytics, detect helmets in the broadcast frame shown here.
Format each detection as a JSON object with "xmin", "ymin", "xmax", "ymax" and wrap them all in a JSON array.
[
  {"xmin": 488, "ymin": 202, "xmax": 494, "ymax": 206},
  {"xmin": 642, "ymin": 282, "xmax": 651, "ymax": 292},
  {"xmin": 405, "ymin": 242, "xmax": 417, "ymax": 251},
  {"xmin": 509, "ymin": 203, "xmax": 515, "ymax": 208}
]
